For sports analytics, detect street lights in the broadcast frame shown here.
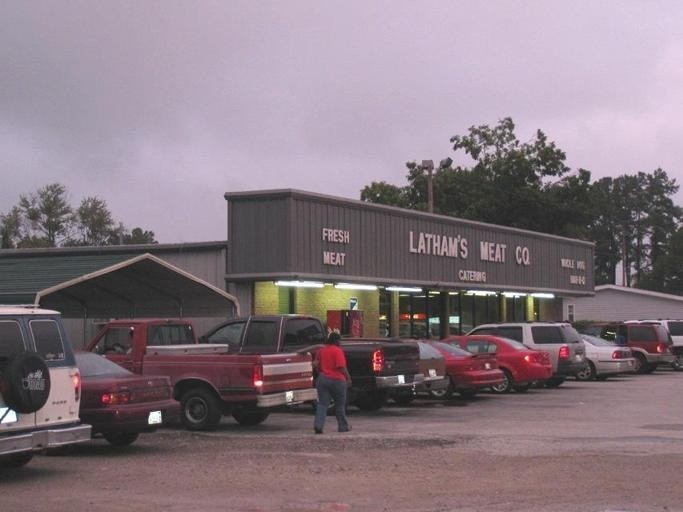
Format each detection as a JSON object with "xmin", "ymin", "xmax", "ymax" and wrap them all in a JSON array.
[{"xmin": 419, "ymin": 157, "xmax": 454, "ymax": 214}]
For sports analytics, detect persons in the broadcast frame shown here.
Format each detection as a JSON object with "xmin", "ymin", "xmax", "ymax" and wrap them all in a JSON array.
[{"xmin": 309, "ymin": 331, "xmax": 353, "ymax": 434}]
